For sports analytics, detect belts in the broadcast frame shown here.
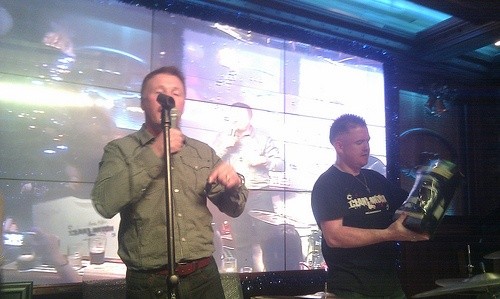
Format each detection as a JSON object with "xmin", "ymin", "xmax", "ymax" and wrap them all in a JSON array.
[{"xmin": 136, "ymin": 257, "xmax": 210, "ymax": 276}]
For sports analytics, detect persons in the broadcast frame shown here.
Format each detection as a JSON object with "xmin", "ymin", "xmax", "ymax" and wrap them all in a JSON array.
[
  {"xmin": 311, "ymin": 114, "xmax": 429, "ymax": 299},
  {"xmin": 2, "ymin": 217, "xmax": 82, "ymax": 282},
  {"xmin": 213, "ymin": 102, "xmax": 286, "ymax": 272},
  {"xmin": 92, "ymin": 66, "xmax": 249, "ymax": 299}
]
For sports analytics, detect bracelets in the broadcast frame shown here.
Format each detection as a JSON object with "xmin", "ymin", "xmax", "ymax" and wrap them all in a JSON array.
[{"xmin": 237, "ymin": 173, "xmax": 245, "ymax": 186}]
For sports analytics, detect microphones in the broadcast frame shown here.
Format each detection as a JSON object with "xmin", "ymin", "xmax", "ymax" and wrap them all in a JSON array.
[{"xmin": 169, "ymin": 108, "xmax": 178, "ymax": 128}]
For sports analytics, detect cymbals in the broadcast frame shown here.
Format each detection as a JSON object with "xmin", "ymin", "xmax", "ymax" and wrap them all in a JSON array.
[
  {"xmin": 410, "ymin": 250, "xmax": 500, "ymax": 299},
  {"xmin": 249, "ymin": 290, "xmax": 335, "ymax": 299}
]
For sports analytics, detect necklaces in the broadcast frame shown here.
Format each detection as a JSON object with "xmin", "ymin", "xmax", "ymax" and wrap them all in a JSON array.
[{"xmin": 352, "ymin": 173, "xmax": 371, "ymax": 193}]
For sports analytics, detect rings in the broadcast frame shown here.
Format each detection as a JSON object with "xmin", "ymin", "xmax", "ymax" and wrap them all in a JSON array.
[{"xmin": 226, "ymin": 175, "xmax": 230, "ymax": 180}]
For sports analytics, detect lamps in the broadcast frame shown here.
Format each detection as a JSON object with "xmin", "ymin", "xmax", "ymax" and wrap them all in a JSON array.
[{"xmin": 422, "ymin": 84, "xmax": 447, "ymax": 114}]
